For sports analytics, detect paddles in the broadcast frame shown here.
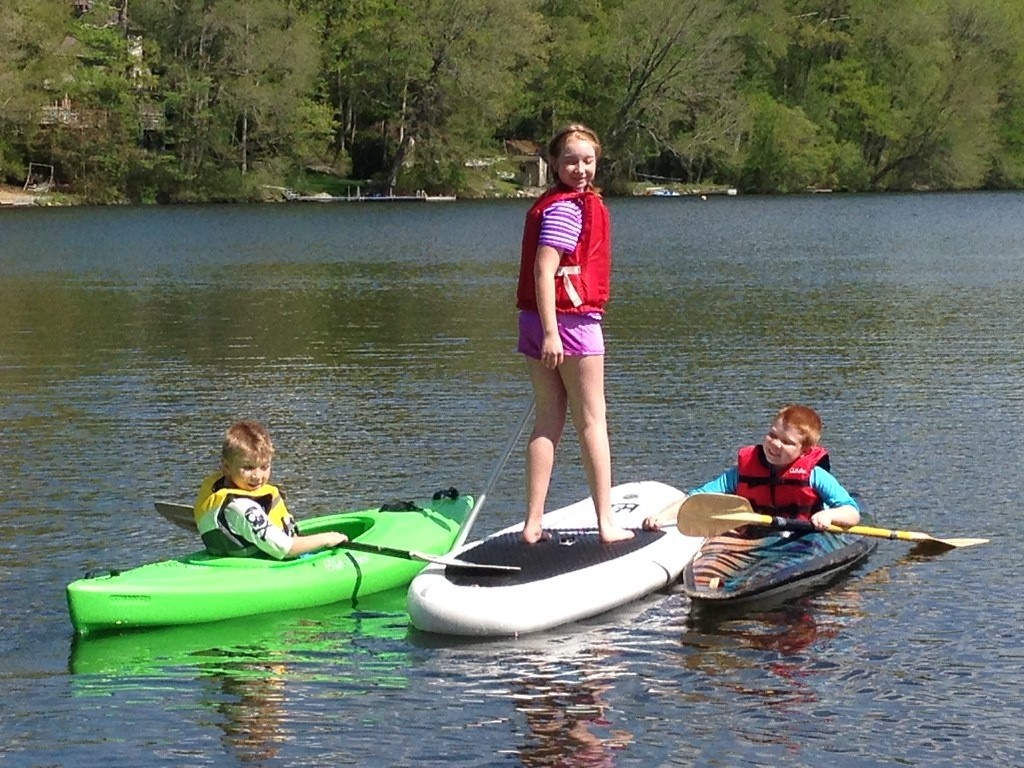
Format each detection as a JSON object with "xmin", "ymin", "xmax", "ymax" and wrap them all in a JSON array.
[
  {"xmin": 153, "ymin": 500, "xmax": 520, "ymax": 573},
  {"xmin": 676, "ymin": 491, "xmax": 991, "ymax": 549},
  {"xmin": 451, "ymin": 395, "xmax": 539, "ymax": 550}
]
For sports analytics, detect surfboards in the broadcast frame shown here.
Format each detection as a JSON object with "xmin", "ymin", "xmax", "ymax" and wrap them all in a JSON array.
[{"xmin": 405, "ymin": 479, "xmax": 703, "ymax": 638}]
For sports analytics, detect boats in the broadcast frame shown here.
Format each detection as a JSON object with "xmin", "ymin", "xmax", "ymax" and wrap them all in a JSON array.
[
  {"xmin": 685, "ymin": 495, "xmax": 877, "ymax": 611},
  {"xmin": 65, "ymin": 495, "xmax": 474, "ymax": 626}
]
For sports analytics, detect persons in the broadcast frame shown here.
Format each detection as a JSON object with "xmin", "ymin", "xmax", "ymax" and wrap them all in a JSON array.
[
  {"xmin": 642, "ymin": 406, "xmax": 861, "ymax": 531},
  {"xmin": 195, "ymin": 421, "xmax": 349, "ymax": 560},
  {"xmin": 517, "ymin": 125, "xmax": 636, "ymax": 544}
]
